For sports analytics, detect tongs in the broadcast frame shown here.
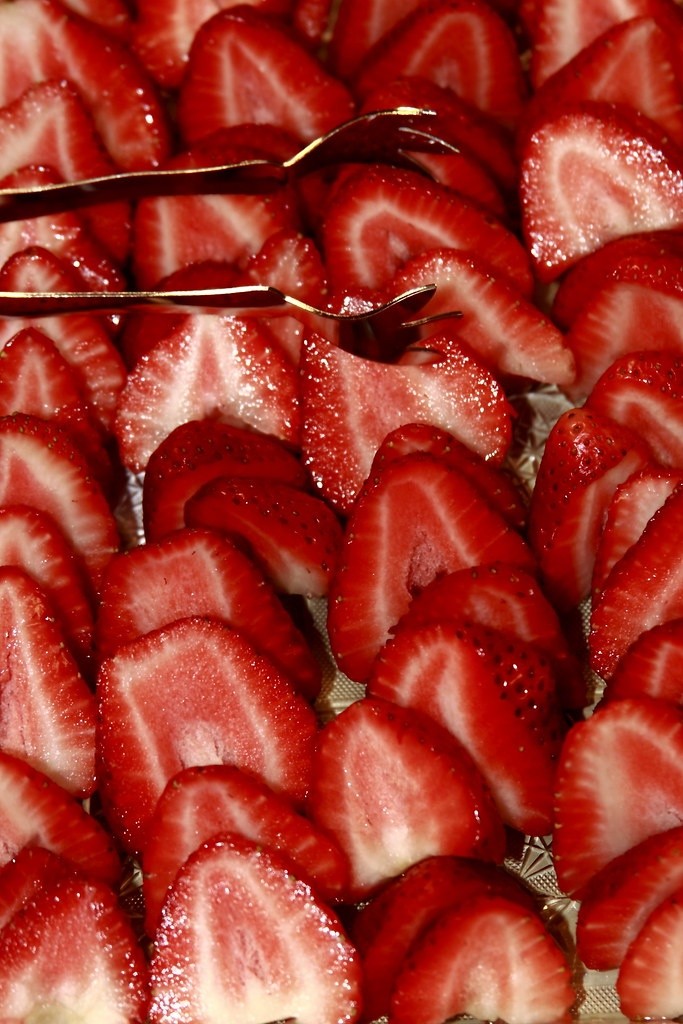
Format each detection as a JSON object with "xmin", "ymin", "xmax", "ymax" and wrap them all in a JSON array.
[{"xmin": 0, "ymin": 107, "xmax": 467, "ymax": 364}]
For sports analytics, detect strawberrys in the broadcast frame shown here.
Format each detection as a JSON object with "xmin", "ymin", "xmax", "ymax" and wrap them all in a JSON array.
[{"xmin": 0, "ymin": 0, "xmax": 683, "ymax": 1024}]
